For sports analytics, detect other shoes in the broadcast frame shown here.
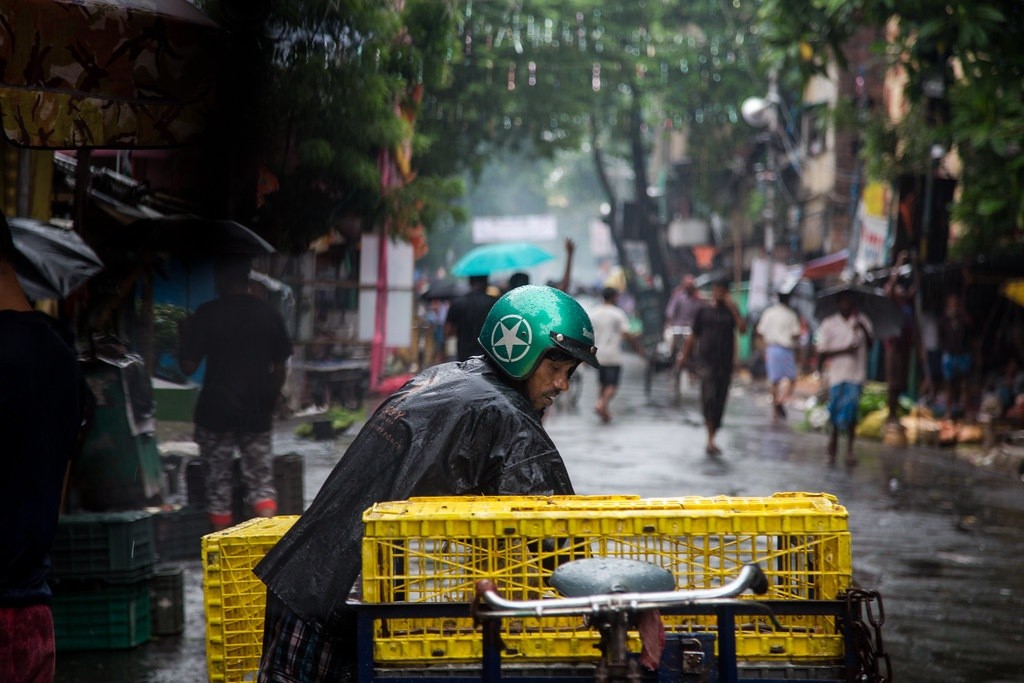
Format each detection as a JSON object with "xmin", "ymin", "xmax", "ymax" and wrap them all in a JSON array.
[
  {"xmin": 705, "ymin": 443, "xmax": 720, "ymax": 455},
  {"xmin": 595, "ymin": 404, "xmax": 609, "ymax": 423},
  {"xmin": 776, "ymin": 403, "xmax": 786, "ymax": 419}
]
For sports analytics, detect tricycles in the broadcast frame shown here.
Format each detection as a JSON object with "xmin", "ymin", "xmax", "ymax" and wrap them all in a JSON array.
[{"xmin": 471, "ymin": 558, "xmax": 781, "ymax": 683}]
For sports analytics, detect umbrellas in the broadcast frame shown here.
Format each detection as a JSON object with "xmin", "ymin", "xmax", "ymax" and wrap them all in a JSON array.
[
  {"xmin": 420, "ymin": 281, "xmax": 467, "ymax": 299},
  {"xmin": 449, "ymin": 241, "xmax": 555, "ymax": 279}
]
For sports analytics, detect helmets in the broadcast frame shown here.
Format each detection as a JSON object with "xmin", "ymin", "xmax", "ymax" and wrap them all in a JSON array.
[{"xmin": 477, "ymin": 284, "xmax": 602, "ymax": 380}]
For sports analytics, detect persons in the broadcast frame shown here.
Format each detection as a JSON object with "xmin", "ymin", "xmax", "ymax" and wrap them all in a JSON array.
[
  {"xmin": 940, "ymin": 295, "xmax": 978, "ymax": 421},
  {"xmin": 666, "ymin": 275, "xmax": 704, "ymax": 321},
  {"xmin": 812, "ymin": 290, "xmax": 865, "ymax": 465},
  {"xmin": 511, "ymin": 238, "xmax": 575, "ymax": 292},
  {"xmin": 678, "ymin": 280, "xmax": 747, "ymax": 455},
  {"xmin": 252, "ymin": 284, "xmax": 601, "ymax": 683},
  {"xmin": 175, "ymin": 263, "xmax": 296, "ymax": 530},
  {"xmin": 587, "ymin": 287, "xmax": 648, "ymax": 423},
  {"xmin": 0, "ymin": 210, "xmax": 81, "ymax": 683},
  {"xmin": 444, "ymin": 276, "xmax": 497, "ymax": 362},
  {"xmin": 883, "ymin": 251, "xmax": 915, "ymax": 424},
  {"xmin": 756, "ymin": 291, "xmax": 802, "ymax": 419},
  {"xmin": 426, "ymin": 299, "xmax": 448, "ymax": 363}
]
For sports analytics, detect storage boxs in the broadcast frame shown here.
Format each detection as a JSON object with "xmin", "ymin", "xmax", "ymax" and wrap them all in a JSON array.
[
  {"xmin": 47, "ymin": 504, "xmax": 209, "ymax": 646},
  {"xmin": 364, "ymin": 493, "xmax": 853, "ymax": 664},
  {"xmin": 201, "ymin": 514, "xmax": 302, "ymax": 683}
]
